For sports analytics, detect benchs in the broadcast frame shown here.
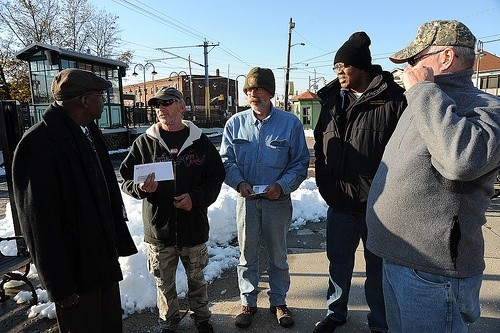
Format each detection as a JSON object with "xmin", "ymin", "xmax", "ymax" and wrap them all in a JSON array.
[{"xmin": 0, "ymin": 236, "xmax": 38, "ymax": 304}]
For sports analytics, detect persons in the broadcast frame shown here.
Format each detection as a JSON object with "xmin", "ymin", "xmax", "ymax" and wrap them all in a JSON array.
[
  {"xmin": 219, "ymin": 68, "xmax": 310, "ymax": 328},
  {"xmin": 12, "ymin": 69, "xmax": 138, "ymax": 333},
  {"xmin": 365, "ymin": 20, "xmax": 500, "ymax": 333},
  {"xmin": 118, "ymin": 86, "xmax": 226, "ymax": 333},
  {"xmin": 311, "ymin": 31, "xmax": 409, "ymax": 333}
]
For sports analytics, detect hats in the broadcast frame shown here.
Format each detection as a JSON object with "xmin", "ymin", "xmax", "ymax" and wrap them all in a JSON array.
[
  {"xmin": 147, "ymin": 86, "xmax": 183, "ymax": 106},
  {"xmin": 333, "ymin": 31, "xmax": 374, "ymax": 73},
  {"xmin": 51, "ymin": 68, "xmax": 113, "ymax": 101},
  {"xmin": 243, "ymin": 67, "xmax": 276, "ymax": 99},
  {"xmin": 388, "ymin": 19, "xmax": 477, "ymax": 65}
]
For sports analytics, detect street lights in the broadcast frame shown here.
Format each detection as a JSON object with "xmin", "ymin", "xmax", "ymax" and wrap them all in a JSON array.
[
  {"xmin": 168, "ymin": 71, "xmax": 189, "ymax": 91},
  {"xmin": 475, "ymin": 40, "xmax": 486, "ymax": 88},
  {"xmin": 132, "ymin": 62, "xmax": 158, "ymax": 127},
  {"xmin": 284, "ymin": 42, "xmax": 306, "ymax": 111}
]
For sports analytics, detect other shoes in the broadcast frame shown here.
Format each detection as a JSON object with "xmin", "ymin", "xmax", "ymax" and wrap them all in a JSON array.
[
  {"xmin": 196, "ymin": 320, "xmax": 214, "ymax": 333},
  {"xmin": 371, "ymin": 330, "xmax": 388, "ymax": 333},
  {"xmin": 234, "ymin": 305, "xmax": 258, "ymax": 328},
  {"xmin": 311, "ymin": 317, "xmax": 347, "ymax": 333},
  {"xmin": 159, "ymin": 329, "xmax": 175, "ymax": 333},
  {"xmin": 270, "ymin": 304, "xmax": 296, "ymax": 328}
]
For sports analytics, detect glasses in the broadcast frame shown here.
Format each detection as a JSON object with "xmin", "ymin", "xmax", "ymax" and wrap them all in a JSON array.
[
  {"xmin": 152, "ymin": 99, "xmax": 176, "ymax": 109},
  {"xmin": 407, "ymin": 50, "xmax": 459, "ymax": 67},
  {"xmin": 246, "ymin": 87, "xmax": 266, "ymax": 96},
  {"xmin": 88, "ymin": 92, "xmax": 110, "ymax": 103},
  {"xmin": 333, "ymin": 64, "xmax": 350, "ymax": 73}
]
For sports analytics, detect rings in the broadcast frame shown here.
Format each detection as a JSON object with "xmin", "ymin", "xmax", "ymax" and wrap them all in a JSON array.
[{"xmin": 270, "ymin": 196, "xmax": 271, "ymax": 199}]
